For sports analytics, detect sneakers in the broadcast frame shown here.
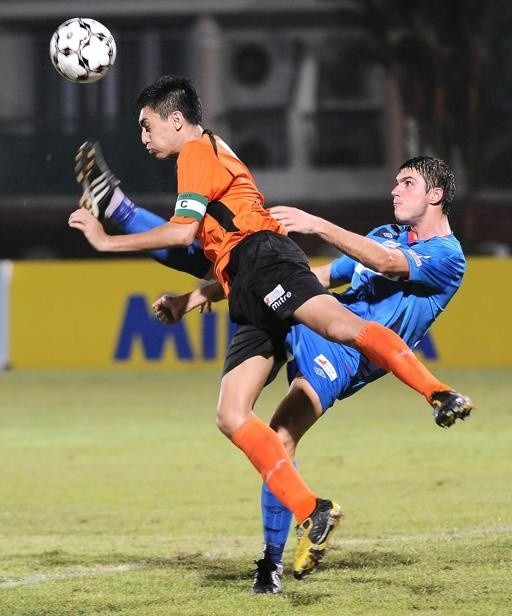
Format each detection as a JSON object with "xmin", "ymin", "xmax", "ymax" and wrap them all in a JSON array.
[
  {"xmin": 430, "ymin": 390, "xmax": 475, "ymax": 428},
  {"xmin": 290, "ymin": 497, "xmax": 345, "ymax": 581},
  {"xmin": 73, "ymin": 138, "xmax": 121, "ymax": 225},
  {"xmin": 251, "ymin": 559, "xmax": 283, "ymax": 596}
]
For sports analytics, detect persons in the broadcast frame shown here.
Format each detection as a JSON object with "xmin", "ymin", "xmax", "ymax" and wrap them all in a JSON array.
[
  {"xmin": 68, "ymin": 74, "xmax": 472, "ymax": 582},
  {"xmin": 73, "ymin": 137, "xmax": 466, "ymax": 592}
]
